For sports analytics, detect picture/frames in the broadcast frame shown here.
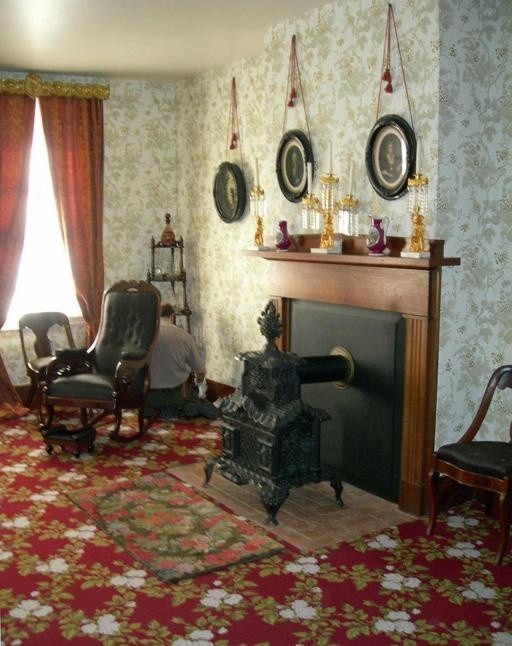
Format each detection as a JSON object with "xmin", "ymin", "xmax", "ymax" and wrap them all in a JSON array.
[
  {"xmin": 213, "ymin": 162, "xmax": 247, "ymax": 223},
  {"xmin": 365, "ymin": 112, "xmax": 415, "ymax": 199},
  {"xmin": 275, "ymin": 129, "xmax": 314, "ymax": 203}
]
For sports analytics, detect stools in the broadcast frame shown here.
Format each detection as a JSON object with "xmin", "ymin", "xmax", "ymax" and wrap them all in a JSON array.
[{"xmin": 39, "ymin": 427, "xmax": 96, "ymax": 459}]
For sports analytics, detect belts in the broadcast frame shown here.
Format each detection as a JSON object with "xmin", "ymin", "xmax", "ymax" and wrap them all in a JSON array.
[{"xmin": 149, "ymin": 386, "xmax": 180, "ymax": 393}]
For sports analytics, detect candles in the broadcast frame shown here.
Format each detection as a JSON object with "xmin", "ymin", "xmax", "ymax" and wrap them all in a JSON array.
[
  {"xmin": 251, "ymin": 151, "xmax": 260, "ymax": 188},
  {"xmin": 327, "ymin": 140, "xmax": 333, "ymax": 177},
  {"xmin": 414, "ymin": 138, "xmax": 421, "ymax": 182},
  {"xmin": 348, "ymin": 160, "xmax": 354, "ymax": 196},
  {"xmin": 307, "ymin": 160, "xmax": 313, "ymax": 197}
]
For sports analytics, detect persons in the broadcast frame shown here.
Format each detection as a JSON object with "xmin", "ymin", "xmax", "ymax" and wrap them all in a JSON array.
[
  {"xmin": 378, "ymin": 141, "xmax": 404, "ymax": 184},
  {"xmin": 135, "ymin": 302, "xmax": 226, "ymax": 419},
  {"xmin": 288, "ymin": 152, "xmax": 305, "ymax": 186}
]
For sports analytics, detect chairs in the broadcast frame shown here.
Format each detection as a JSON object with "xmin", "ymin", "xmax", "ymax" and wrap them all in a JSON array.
[
  {"xmin": 41, "ymin": 279, "xmax": 161, "ymax": 443},
  {"xmin": 19, "ymin": 311, "xmax": 76, "ymax": 409},
  {"xmin": 426, "ymin": 365, "xmax": 511, "ymax": 565}
]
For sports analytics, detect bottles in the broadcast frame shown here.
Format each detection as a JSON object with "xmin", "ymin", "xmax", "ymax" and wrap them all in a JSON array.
[{"xmin": 162, "ymin": 213, "xmax": 175, "ymax": 247}]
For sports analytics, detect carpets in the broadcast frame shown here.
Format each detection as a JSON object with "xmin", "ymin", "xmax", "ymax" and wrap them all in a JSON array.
[{"xmin": 64, "ymin": 471, "xmax": 286, "ymax": 586}]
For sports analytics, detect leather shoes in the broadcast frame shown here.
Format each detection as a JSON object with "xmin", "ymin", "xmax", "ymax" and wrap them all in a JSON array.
[
  {"xmin": 183, "ymin": 401, "xmax": 219, "ymax": 419},
  {"xmin": 160, "ymin": 407, "xmax": 185, "ymax": 418}
]
